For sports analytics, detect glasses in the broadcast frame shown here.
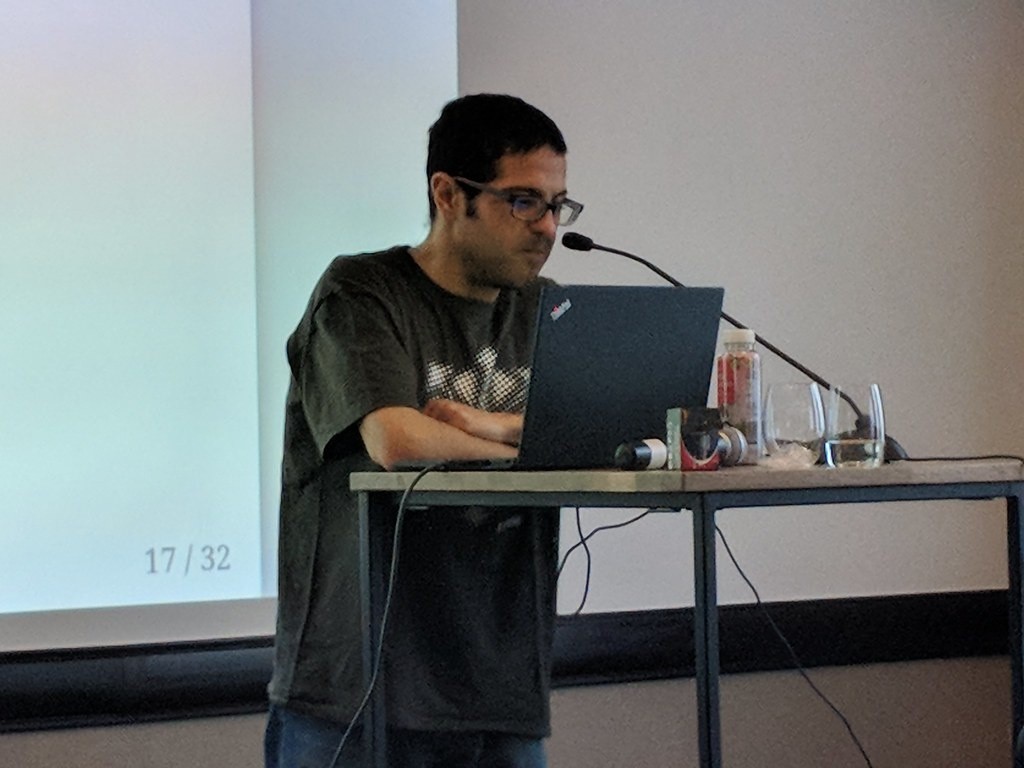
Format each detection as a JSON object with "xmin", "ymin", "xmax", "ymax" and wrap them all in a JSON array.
[{"xmin": 452, "ymin": 176, "xmax": 583, "ymax": 226}]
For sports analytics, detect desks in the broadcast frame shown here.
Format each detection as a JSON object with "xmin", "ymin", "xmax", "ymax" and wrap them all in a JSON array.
[{"xmin": 349, "ymin": 460, "xmax": 1024, "ymax": 768}]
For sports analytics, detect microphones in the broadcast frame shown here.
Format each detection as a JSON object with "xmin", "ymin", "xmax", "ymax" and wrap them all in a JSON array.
[
  {"xmin": 614, "ymin": 427, "xmax": 749, "ymax": 471},
  {"xmin": 562, "ymin": 233, "xmax": 910, "ymax": 463}
]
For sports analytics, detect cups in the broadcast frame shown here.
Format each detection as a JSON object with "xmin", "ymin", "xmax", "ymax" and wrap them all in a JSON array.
[
  {"xmin": 763, "ymin": 381, "xmax": 823, "ymax": 465},
  {"xmin": 825, "ymin": 383, "xmax": 885, "ymax": 469}
]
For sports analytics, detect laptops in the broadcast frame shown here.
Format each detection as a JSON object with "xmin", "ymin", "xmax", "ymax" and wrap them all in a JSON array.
[{"xmin": 387, "ymin": 286, "xmax": 724, "ymax": 473}]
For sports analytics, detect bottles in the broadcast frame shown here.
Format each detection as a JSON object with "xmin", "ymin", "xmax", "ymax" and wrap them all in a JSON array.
[{"xmin": 717, "ymin": 330, "xmax": 762, "ymax": 464}]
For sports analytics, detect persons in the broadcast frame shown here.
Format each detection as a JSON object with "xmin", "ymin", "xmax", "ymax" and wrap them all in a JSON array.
[{"xmin": 263, "ymin": 93, "xmax": 567, "ymax": 768}]
